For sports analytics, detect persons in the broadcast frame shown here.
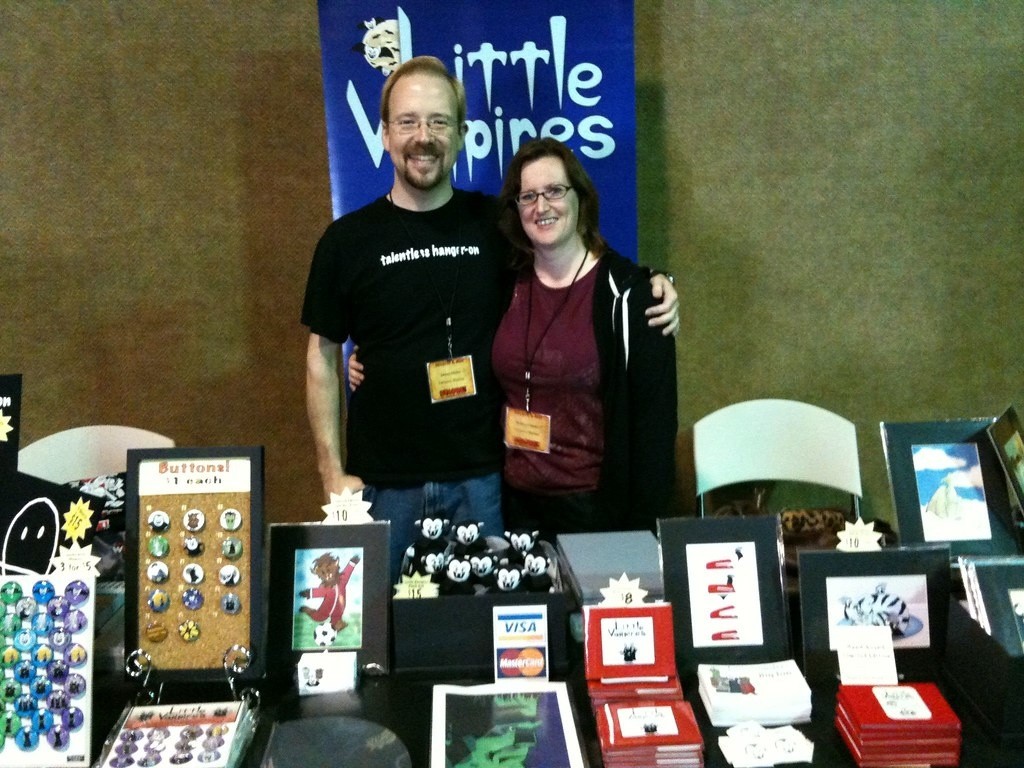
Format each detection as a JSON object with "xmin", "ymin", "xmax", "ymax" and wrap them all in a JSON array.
[
  {"xmin": 299, "ymin": 55, "xmax": 680, "ymax": 579},
  {"xmin": 349, "ymin": 139, "xmax": 684, "ymax": 544}
]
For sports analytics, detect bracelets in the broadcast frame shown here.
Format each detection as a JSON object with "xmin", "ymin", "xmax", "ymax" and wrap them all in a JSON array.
[{"xmin": 653, "ymin": 270, "xmax": 676, "ymax": 287}]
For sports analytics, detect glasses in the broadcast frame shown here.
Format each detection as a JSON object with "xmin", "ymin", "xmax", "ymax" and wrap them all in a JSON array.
[
  {"xmin": 382, "ymin": 114, "xmax": 459, "ymax": 135},
  {"xmin": 514, "ymin": 183, "xmax": 572, "ymax": 205}
]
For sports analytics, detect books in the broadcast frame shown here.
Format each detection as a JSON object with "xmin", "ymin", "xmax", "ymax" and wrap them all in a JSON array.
[
  {"xmin": 556, "ymin": 531, "xmax": 666, "ymax": 606},
  {"xmin": 698, "ymin": 659, "xmax": 812, "ymax": 727}
]
